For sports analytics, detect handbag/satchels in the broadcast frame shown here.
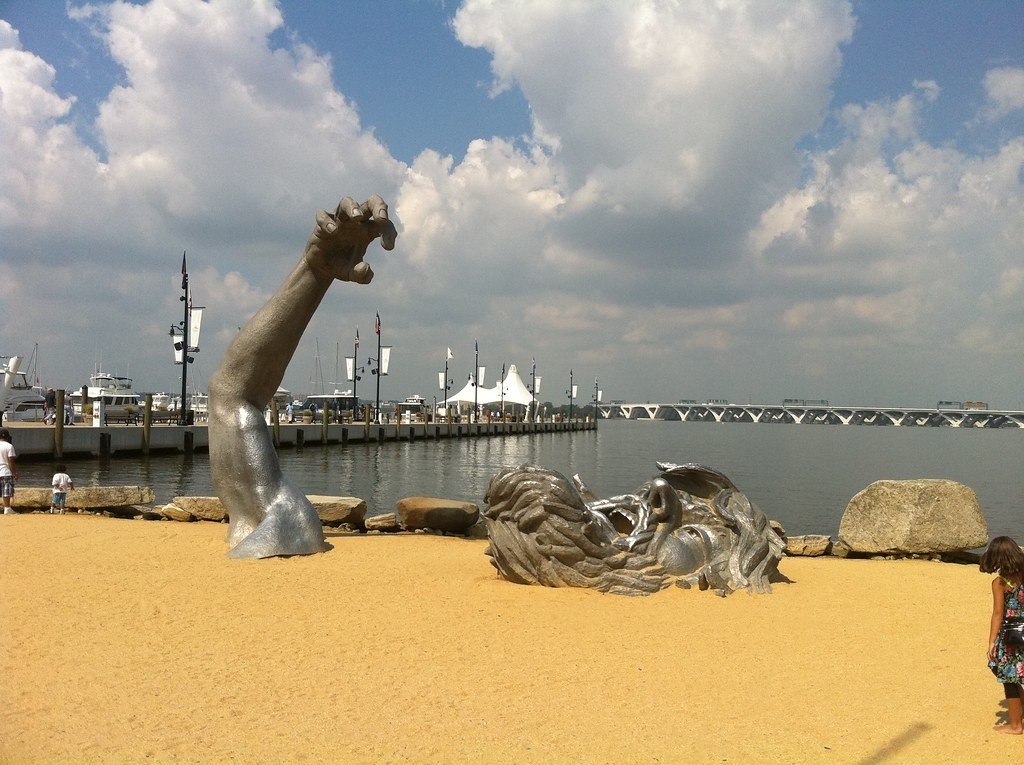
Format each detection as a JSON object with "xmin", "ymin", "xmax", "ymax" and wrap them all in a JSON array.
[{"xmin": 1000, "ymin": 622, "xmax": 1024, "ymax": 645}]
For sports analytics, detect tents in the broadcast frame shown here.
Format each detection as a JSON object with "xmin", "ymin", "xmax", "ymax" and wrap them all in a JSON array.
[{"xmin": 436, "ymin": 364, "xmax": 540, "ymax": 422}]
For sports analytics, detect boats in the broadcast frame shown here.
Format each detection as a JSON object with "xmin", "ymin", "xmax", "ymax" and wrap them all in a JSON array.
[{"xmin": 0, "ymin": 334, "xmax": 433, "ymax": 422}]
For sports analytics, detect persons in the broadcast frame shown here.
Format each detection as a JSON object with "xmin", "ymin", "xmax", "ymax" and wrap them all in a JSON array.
[
  {"xmin": 272, "ymin": 399, "xmax": 319, "ymax": 426},
  {"xmin": 43, "ymin": 388, "xmax": 75, "ymax": 426},
  {"xmin": 485, "ymin": 408, "xmax": 527, "ymax": 423},
  {"xmin": 979, "ymin": 536, "xmax": 1024, "ymax": 735},
  {"xmin": 208, "ymin": 197, "xmax": 796, "ymax": 597},
  {"xmin": 546, "ymin": 410, "xmax": 571, "ymax": 423},
  {"xmin": 393, "ymin": 406, "xmax": 402, "ymax": 421},
  {"xmin": 0, "ymin": 427, "xmax": 18, "ymax": 514},
  {"xmin": 50, "ymin": 465, "xmax": 75, "ymax": 515},
  {"xmin": 354, "ymin": 403, "xmax": 375, "ymax": 422}
]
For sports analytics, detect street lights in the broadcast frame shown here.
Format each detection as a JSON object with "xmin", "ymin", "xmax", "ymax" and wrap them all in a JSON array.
[
  {"xmin": 353, "ymin": 337, "xmax": 365, "ymax": 421},
  {"xmin": 468, "ymin": 338, "xmax": 478, "ymax": 423},
  {"xmin": 526, "ymin": 356, "xmax": 535, "ymax": 422},
  {"xmin": 444, "ymin": 357, "xmax": 454, "ymax": 409},
  {"xmin": 500, "ymin": 361, "xmax": 508, "ymax": 418},
  {"xmin": 169, "ymin": 250, "xmax": 194, "ymax": 425},
  {"xmin": 367, "ymin": 309, "xmax": 380, "ymax": 424},
  {"xmin": 591, "ymin": 377, "xmax": 598, "ymax": 421},
  {"xmin": 565, "ymin": 369, "xmax": 573, "ymax": 418}
]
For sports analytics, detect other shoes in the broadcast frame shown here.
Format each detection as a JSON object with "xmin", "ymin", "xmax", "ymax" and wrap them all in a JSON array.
[
  {"xmin": 42, "ymin": 417, "xmax": 47, "ymax": 425},
  {"xmin": 50, "ymin": 507, "xmax": 54, "ymax": 513},
  {"xmin": 59, "ymin": 508, "xmax": 65, "ymax": 514},
  {"xmin": 69, "ymin": 423, "xmax": 75, "ymax": 425},
  {"xmin": 4, "ymin": 507, "xmax": 16, "ymax": 514}
]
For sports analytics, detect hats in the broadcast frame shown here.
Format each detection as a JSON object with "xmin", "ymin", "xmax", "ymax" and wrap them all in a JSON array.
[{"xmin": 0, "ymin": 428, "xmax": 9, "ymax": 437}]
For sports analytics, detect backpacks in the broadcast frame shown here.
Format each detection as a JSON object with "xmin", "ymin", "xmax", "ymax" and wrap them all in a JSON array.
[{"xmin": 310, "ymin": 404, "xmax": 316, "ymax": 412}]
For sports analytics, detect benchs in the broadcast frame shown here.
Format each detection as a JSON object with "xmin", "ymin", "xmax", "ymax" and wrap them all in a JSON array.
[{"xmin": 104, "ymin": 413, "xmax": 179, "ymax": 426}]
